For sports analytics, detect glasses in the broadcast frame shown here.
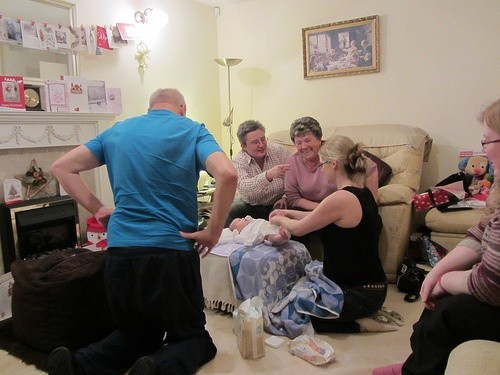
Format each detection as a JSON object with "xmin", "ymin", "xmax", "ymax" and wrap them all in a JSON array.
[
  {"xmin": 480, "ymin": 139, "xmax": 500, "ymax": 147},
  {"xmin": 317, "ymin": 161, "xmax": 330, "ymax": 170}
]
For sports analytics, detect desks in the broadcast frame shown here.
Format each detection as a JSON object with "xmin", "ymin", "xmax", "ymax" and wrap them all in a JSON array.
[{"xmin": 0, "ymin": 112, "xmax": 116, "ymax": 275}]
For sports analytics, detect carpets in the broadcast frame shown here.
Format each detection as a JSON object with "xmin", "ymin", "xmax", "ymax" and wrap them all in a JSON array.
[{"xmin": 0, "ymin": 276, "xmax": 423, "ymax": 375}]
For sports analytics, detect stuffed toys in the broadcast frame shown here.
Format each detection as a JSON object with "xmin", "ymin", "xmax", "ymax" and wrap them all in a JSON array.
[{"xmin": 457, "ymin": 155, "xmax": 492, "ymax": 196}]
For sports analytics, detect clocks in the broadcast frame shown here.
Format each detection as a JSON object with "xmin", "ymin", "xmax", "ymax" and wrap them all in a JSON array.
[{"xmin": 23, "ymin": 84, "xmax": 45, "ymax": 111}]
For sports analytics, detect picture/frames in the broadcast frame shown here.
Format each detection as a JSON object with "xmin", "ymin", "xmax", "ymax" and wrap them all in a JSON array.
[{"xmin": 302, "ymin": 15, "xmax": 380, "ymax": 80}]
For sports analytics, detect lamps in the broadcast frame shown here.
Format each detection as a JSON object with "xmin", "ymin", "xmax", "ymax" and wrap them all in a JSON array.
[
  {"xmin": 212, "ymin": 40, "xmax": 251, "ymax": 160},
  {"xmin": 133, "ymin": 7, "xmax": 168, "ymax": 71}
]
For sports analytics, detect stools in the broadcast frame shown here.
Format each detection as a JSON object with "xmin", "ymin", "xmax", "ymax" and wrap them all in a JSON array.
[
  {"xmin": 10, "ymin": 245, "xmax": 106, "ymax": 352},
  {"xmin": 200, "ymin": 239, "xmax": 311, "ymax": 320}
]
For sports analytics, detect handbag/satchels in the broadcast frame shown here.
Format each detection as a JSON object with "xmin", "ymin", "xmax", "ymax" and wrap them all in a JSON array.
[
  {"xmin": 11, "ymin": 248, "xmax": 118, "ymax": 354},
  {"xmin": 396, "ymin": 258, "xmax": 429, "ymax": 302}
]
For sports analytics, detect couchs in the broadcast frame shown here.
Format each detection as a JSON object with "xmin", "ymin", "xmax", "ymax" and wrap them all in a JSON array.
[
  {"xmin": 267, "ymin": 123, "xmax": 433, "ymax": 282},
  {"xmin": 444, "ymin": 340, "xmax": 500, "ymax": 375},
  {"xmin": 424, "ymin": 207, "xmax": 482, "ymax": 258}
]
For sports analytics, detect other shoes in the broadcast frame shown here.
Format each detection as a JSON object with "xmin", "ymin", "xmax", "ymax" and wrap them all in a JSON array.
[
  {"xmin": 371, "ymin": 363, "xmax": 404, "ymax": 375},
  {"xmin": 377, "ymin": 306, "xmax": 405, "ymax": 326},
  {"xmin": 127, "ymin": 357, "xmax": 158, "ymax": 375},
  {"xmin": 47, "ymin": 347, "xmax": 76, "ymax": 375}
]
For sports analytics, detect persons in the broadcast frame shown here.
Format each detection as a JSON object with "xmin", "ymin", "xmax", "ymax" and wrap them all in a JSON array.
[
  {"xmin": 229, "ymin": 217, "xmax": 292, "ymax": 246},
  {"xmin": 310, "ymin": 39, "xmax": 372, "ymax": 72},
  {"xmin": 285, "ymin": 115, "xmax": 379, "ymax": 248},
  {"xmin": 269, "ymin": 133, "xmax": 397, "ymax": 332},
  {"xmin": 224, "ymin": 120, "xmax": 292, "ymax": 228},
  {"xmin": 371, "ymin": 98, "xmax": 500, "ymax": 375},
  {"xmin": 48, "ymin": 88, "xmax": 239, "ymax": 375}
]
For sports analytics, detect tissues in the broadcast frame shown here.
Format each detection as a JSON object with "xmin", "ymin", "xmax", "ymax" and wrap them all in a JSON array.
[{"xmin": 287, "ymin": 335, "xmax": 337, "ymax": 366}]
